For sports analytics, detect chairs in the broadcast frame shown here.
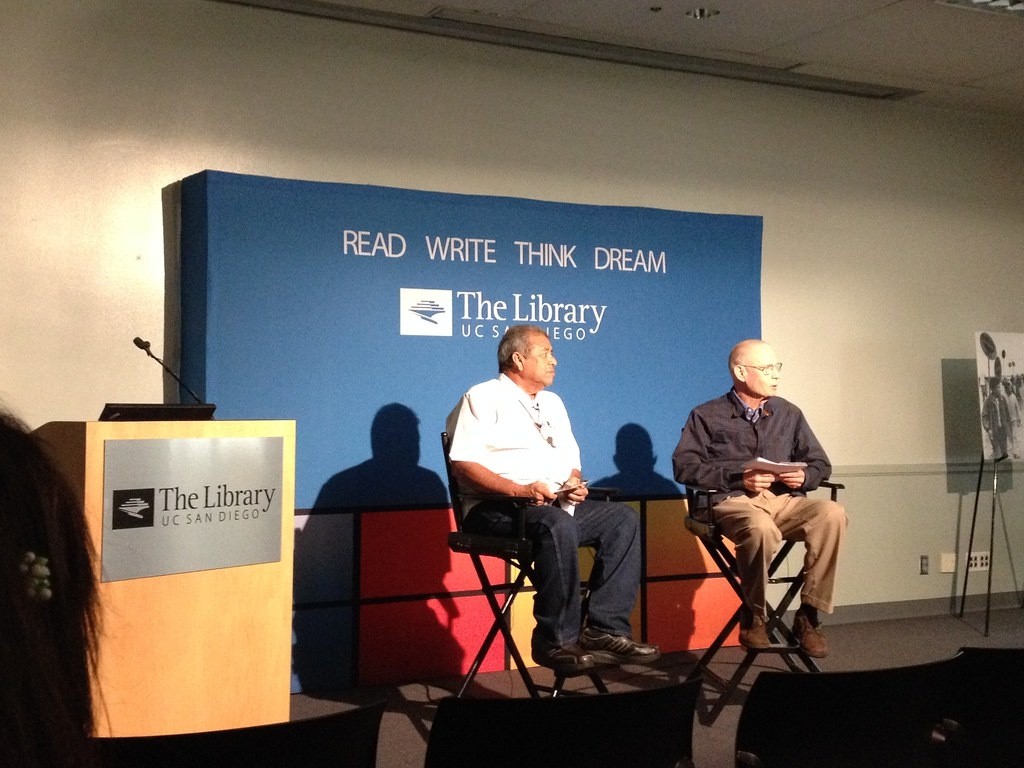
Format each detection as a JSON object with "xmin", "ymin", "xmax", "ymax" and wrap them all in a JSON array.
[
  {"xmin": 680, "ymin": 424, "xmax": 845, "ymax": 723},
  {"xmin": 439, "ymin": 432, "xmax": 609, "ymax": 699}
]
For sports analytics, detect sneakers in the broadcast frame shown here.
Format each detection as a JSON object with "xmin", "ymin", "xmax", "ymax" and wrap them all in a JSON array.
[
  {"xmin": 578, "ymin": 625, "xmax": 664, "ymax": 664},
  {"xmin": 527, "ymin": 638, "xmax": 596, "ymax": 672}
]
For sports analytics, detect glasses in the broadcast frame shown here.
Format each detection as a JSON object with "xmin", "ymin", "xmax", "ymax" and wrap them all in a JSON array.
[{"xmin": 740, "ymin": 363, "xmax": 784, "ymax": 374}]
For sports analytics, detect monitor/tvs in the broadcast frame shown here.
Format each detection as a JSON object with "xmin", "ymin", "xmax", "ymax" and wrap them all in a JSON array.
[{"xmin": 98, "ymin": 403, "xmax": 216, "ymax": 421}]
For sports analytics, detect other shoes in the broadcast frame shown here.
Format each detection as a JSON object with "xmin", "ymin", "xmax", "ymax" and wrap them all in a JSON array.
[
  {"xmin": 737, "ymin": 611, "xmax": 770, "ymax": 650},
  {"xmin": 792, "ymin": 611, "xmax": 830, "ymax": 658}
]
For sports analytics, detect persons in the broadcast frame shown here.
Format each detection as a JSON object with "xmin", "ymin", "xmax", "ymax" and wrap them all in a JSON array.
[
  {"xmin": 672, "ymin": 338, "xmax": 850, "ymax": 659},
  {"xmin": 981, "ymin": 374, "xmax": 1024, "ymax": 460},
  {"xmin": 445, "ymin": 325, "xmax": 661, "ymax": 672}
]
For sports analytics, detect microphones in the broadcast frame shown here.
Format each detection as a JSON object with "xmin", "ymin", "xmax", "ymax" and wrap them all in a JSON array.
[{"xmin": 133, "ymin": 338, "xmax": 203, "ymax": 404}]
